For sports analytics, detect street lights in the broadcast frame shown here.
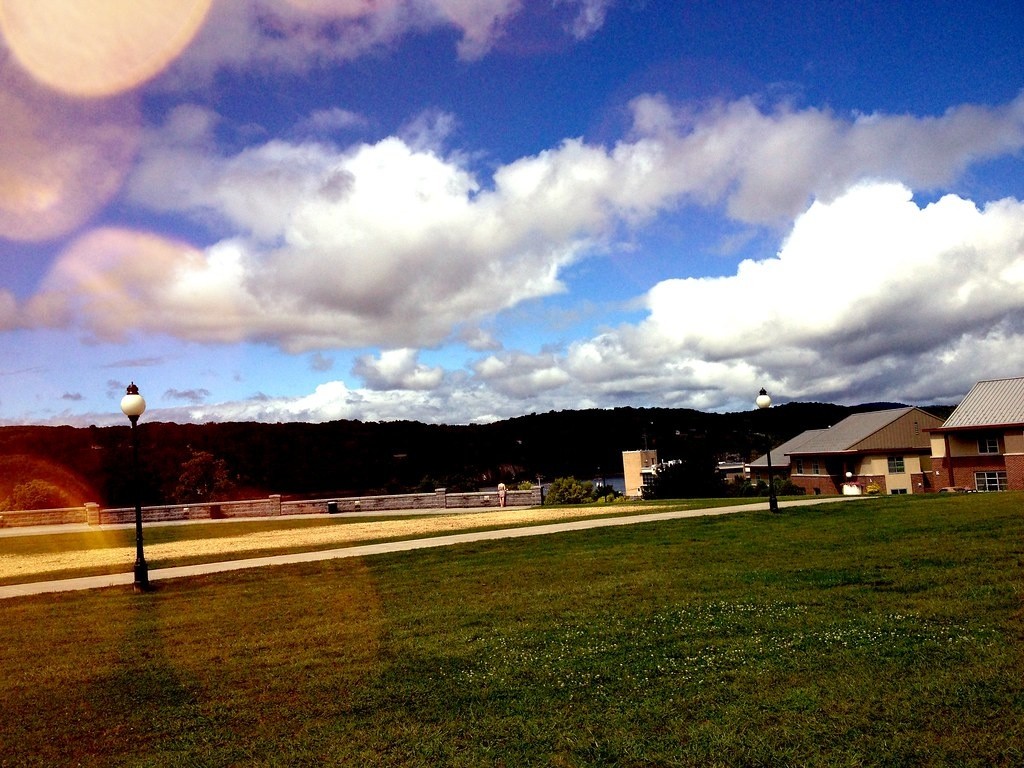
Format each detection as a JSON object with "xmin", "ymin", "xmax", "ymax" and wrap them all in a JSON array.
[
  {"xmin": 120, "ymin": 382, "xmax": 151, "ymax": 595},
  {"xmin": 756, "ymin": 387, "xmax": 778, "ymax": 512}
]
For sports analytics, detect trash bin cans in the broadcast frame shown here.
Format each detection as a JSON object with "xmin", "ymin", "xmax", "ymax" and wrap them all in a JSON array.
[
  {"xmin": 209, "ymin": 505, "xmax": 221, "ymax": 519},
  {"xmin": 327, "ymin": 500, "xmax": 338, "ymax": 514}
]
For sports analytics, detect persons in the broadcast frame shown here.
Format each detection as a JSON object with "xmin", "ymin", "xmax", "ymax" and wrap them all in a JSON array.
[{"xmin": 498, "ymin": 481, "xmax": 506, "ymax": 506}]
